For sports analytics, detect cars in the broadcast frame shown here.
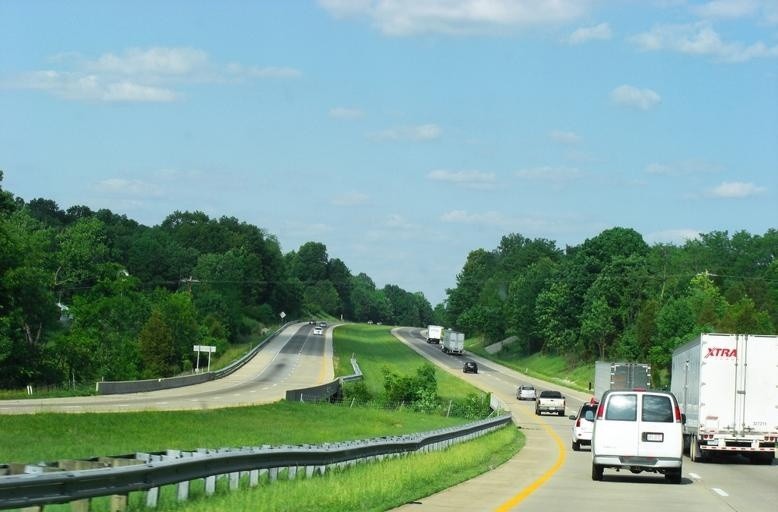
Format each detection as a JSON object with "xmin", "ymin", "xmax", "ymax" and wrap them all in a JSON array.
[
  {"xmin": 517, "ymin": 384, "xmax": 686, "ymax": 481},
  {"xmin": 309, "ymin": 319, "xmax": 327, "ymax": 335}
]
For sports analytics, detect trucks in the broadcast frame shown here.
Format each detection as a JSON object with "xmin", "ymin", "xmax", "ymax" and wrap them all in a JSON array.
[
  {"xmin": 594, "ymin": 333, "xmax": 778, "ymax": 462},
  {"xmin": 425, "ymin": 325, "xmax": 465, "ymax": 355}
]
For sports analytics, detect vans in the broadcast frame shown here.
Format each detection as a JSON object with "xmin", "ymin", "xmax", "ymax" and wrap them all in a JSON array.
[{"xmin": 463, "ymin": 362, "xmax": 477, "ymax": 373}]
